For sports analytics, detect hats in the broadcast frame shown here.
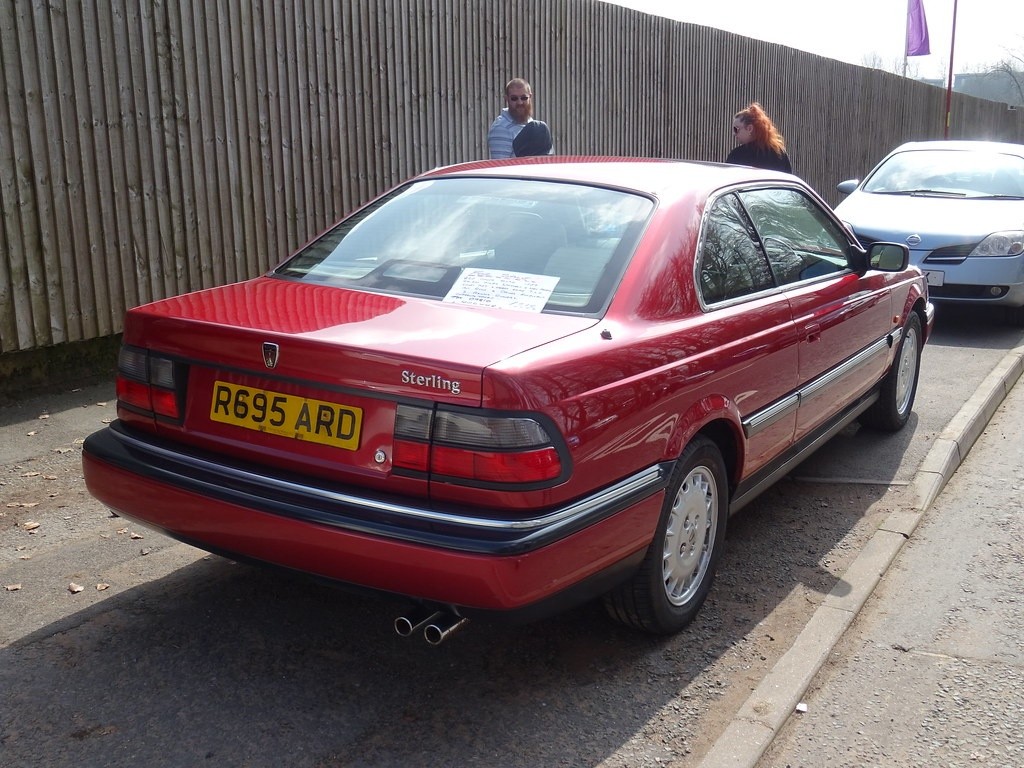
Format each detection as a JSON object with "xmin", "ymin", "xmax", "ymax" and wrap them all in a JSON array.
[{"xmin": 513, "ymin": 120, "xmax": 552, "ymax": 157}]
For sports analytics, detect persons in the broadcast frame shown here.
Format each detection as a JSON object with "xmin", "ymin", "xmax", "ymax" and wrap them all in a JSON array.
[
  {"xmin": 487, "ymin": 77, "xmax": 555, "ymax": 158},
  {"xmin": 724, "ymin": 101, "xmax": 792, "ymax": 176}
]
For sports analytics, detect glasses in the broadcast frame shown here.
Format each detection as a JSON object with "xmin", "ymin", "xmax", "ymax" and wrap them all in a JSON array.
[
  {"xmin": 508, "ymin": 95, "xmax": 530, "ymax": 101},
  {"xmin": 734, "ymin": 127, "xmax": 745, "ymax": 133}
]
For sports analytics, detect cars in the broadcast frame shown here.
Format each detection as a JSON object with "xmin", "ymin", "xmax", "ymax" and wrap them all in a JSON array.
[
  {"xmin": 832, "ymin": 139, "xmax": 1024, "ymax": 330},
  {"xmin": 81, "ymin": 155, "xmax": 935, "ymax": 641}
]
